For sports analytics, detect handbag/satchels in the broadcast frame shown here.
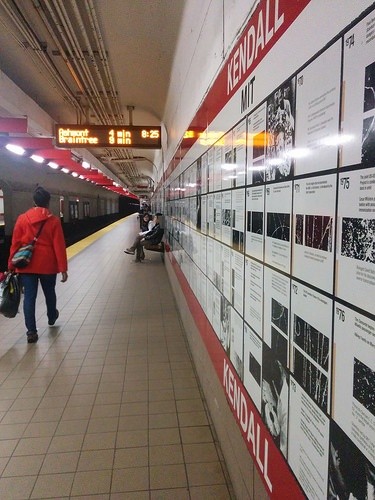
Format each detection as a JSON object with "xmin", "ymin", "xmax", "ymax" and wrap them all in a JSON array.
[{"xmin": 11, "ymin": 244, "xmax": 34, "ymax": 268}]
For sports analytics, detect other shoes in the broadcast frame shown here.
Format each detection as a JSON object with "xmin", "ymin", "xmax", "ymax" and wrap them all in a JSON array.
[{"xmin": 124, "ymin": 249, "xmax": 135, "ymax": 255}]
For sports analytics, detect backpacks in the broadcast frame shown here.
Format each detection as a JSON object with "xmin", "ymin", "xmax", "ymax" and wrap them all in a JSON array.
[{"xmin": 0, "ymin": 272, "xmax": 20, "ymax": 319}]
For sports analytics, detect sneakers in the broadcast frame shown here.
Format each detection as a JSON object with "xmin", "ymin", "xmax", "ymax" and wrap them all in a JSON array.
[
  {"xmin": 27, "ymin": 333, "xmax": 38, "ymax": 343},
  {"xmin": 48, "ymin": 309, "xmax": 59, "ymax": 327}
]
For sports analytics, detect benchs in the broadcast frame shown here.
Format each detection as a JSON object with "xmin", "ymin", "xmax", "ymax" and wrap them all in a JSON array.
[{"xmin": 144, "ymin": 244, "xmax": 164, "ymax": 252}]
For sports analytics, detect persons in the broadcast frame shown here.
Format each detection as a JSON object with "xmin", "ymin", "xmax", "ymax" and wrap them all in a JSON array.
[
  {"xmin": 137, "ymin": 213, "xmax": 157, "ymax": 260},
  {"xmin": 274, "ymin": 84, "xmax": 292, "ymax": 118},
  {"xmin": 329, "ymin": 433, "xmax": 353, "ymax": 487},
  {"xmin": 123, "ymin": 213, "xmax": 165, "ymax": 264},
  {"xmin": 7, "ymin": 187, "xmax": 68, "ymax": 343}
]
